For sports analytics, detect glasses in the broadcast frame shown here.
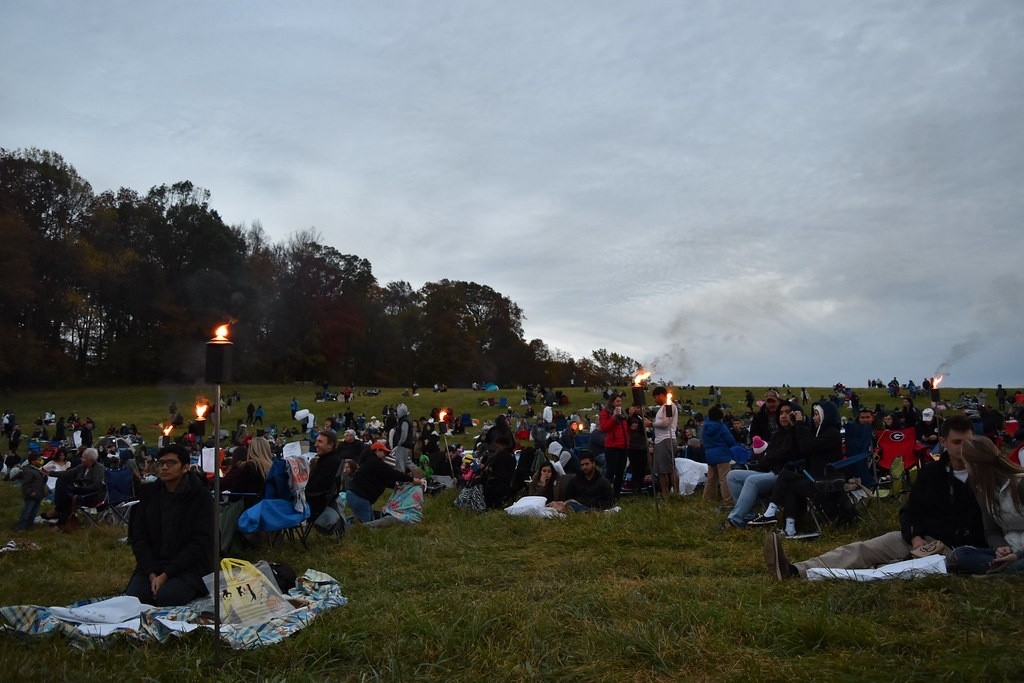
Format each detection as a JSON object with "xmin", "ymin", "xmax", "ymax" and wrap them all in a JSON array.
[
  {"xmin": 155, "ymin": 460, "xmax": 182, "ymax": 468},
  {"xmin": 857, "ymin": 407, "xmax": 873, "ymax": 413}
]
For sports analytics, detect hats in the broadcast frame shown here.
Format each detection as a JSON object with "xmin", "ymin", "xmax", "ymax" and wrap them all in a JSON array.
[
  {"xmin": 763, "ymin": 390, "xmax": 780, "ymax": 402},
  {"xmin": 370, "ymin": 416, "xmax": 376, "ymax": 420},
  {"xmin": 370, "ymin": 442, "xmax": 391, "ymax": 452},
  {"xmin": 922, "ymin": 408, "xmax": 934, "ymax": 422},
  {"xmin": 751, "ymin": 436, "xmax": 769, "ymax": 454},
  {"xmin": 463, "ymin": 454, "xmax": 473, "ymax": 464},
  {"xmin": 911, "ymin": 540, "xmax": 945, "ymax": 558}
]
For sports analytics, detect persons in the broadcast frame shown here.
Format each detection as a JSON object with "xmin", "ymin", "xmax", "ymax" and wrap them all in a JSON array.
[{"xmin": 0, "ymin": 377, "xmax": 1024, "ymax": 607}]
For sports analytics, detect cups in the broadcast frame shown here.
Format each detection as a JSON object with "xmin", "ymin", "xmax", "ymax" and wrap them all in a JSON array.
[{"xmin": 616, "ymin": 407, "xmax": 621, "ymax": 414}]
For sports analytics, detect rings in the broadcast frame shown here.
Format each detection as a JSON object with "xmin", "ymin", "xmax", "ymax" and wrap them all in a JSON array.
[{"xmin": 797, "ymin": 412, "xmax": 799, "ymax": 414}]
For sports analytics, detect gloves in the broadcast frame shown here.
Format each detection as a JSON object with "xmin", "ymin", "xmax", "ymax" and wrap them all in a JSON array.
[{"xmin": 743, "ymin": 458, "xmax": 766, "ymax": 470}]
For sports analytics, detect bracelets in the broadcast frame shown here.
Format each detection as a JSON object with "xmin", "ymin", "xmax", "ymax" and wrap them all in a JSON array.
[{"xmin": 650, "ymin": 421, "xmax": 653, "ymax": 428}]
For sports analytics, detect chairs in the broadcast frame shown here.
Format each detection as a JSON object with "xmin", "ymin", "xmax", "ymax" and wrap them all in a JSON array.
[
  {"xmin": 461, "ymin": 413, "xmax": 473, "ymax": 427},
  {"xmin": 238, "ymin": 455, "xmax": 355, "ymax": 554},
  {"xmin": 499, "ymin": 398, "xmax": 507, "ymax": 408},
  {"xmin": 60, "ymin": 467, "xmax": 140, "ymax": 530},
  {"xmin": 803, "ymin": 422, "xmax": 934, "ymax": 536}
]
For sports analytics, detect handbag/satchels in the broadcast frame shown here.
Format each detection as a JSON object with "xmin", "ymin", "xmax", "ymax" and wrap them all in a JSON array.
[
  {"xmin": 201, "ymin": 558, "xmax": 296, "ymax": 623},
  {"xmin": 890, "ymin": 456, "xmax": 905, "ymax": 480},
  {"xmin": 454, "ymin": 478, "xmax": 486, "ymax": 512}
]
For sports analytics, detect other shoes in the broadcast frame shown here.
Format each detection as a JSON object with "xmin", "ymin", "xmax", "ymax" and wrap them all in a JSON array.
[
  {"xmin": 764, "ymin": 532, "xmax": 792, "ymax": 582},
  {"xmin": 17, "ymin": 527, "xmax": 37, "ymax": 533},
  {"xmin": 772, "ymin": 529, "xmax": 797, "ymax": 536},
  {"xmin": 40, "ymin": 512, "xmax": 59, "ymax": 520},
  {"xmin": 720, "ymin": 501, "xmax": 735, "ymax": 508},
  {"xmin": 57, "ymin": 512, "xmax": 69, "ymax": 529},
  {"xmin": 747, "ymin": 512, "xmax": 778, "ymax": 526},
  {"xmin": 970, "ymin": 561, "xmax": 1015, "ymax": 579},
  {"xmin": 718, "ymin": 520, "xmax": 735, "ymax": 531}
]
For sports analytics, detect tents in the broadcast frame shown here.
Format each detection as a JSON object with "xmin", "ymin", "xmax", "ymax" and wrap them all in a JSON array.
[{"xmin": 484, "ymin": 382, "xmax": 500, "ymax": 391}]
